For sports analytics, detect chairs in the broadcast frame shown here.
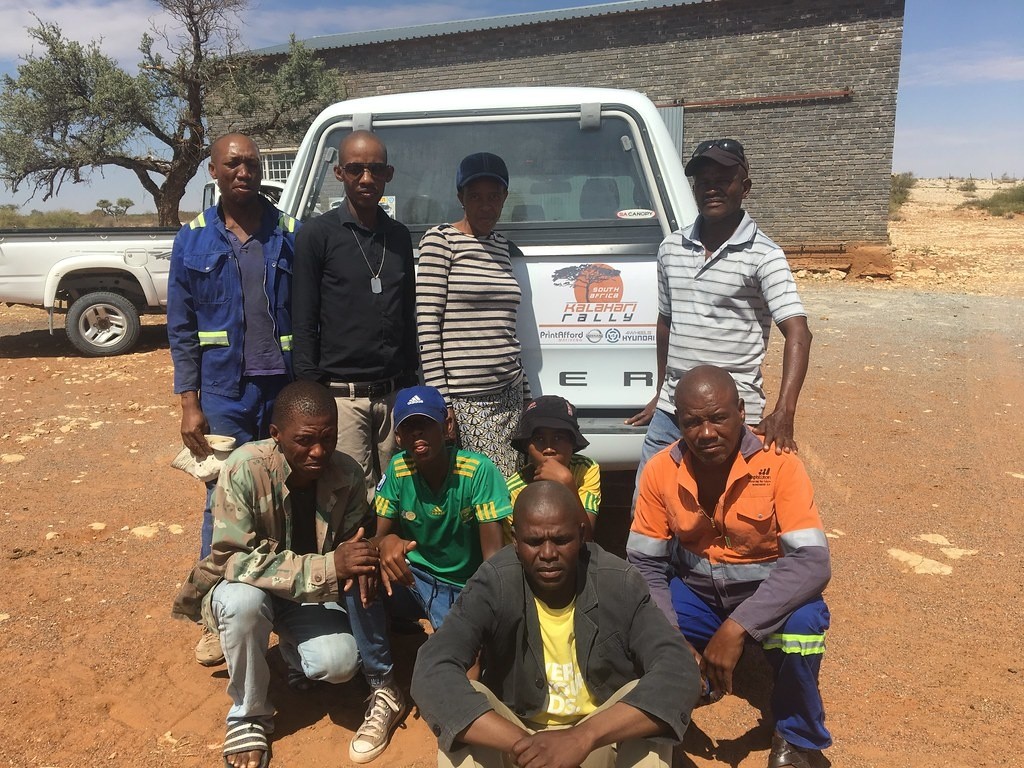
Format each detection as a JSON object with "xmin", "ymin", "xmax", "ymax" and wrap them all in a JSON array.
[
  {"xmin": 577, "ymin": 178, "xmax": 620, "ymax": 218},
  {"xmin": 509, "ymin": 204, "xmax": 544, "ymax": 221},
  {"xmin": 400, "ymin": 192, "xmax": 446, "ymax": 223}
]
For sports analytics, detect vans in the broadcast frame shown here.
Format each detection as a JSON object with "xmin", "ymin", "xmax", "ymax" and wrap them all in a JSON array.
[{"xmin": 277, "ymin": 86, "xmax": 702, "ymax": 511}]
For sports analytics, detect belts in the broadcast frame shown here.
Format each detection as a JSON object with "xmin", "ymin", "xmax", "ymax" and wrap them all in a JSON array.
[{"xmin": 327, "ymin": 371, "xmax": 410, "ymax": 400}]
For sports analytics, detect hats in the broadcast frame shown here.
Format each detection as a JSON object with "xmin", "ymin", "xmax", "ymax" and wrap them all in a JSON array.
[
  {"xmin": 170, "ymin": 434, "xmax": 237, "ymax": 484},
  {"xmin": 392, "ymin": 387, "xmax": 447, "ymax": 432},
  {"xmin": 456, "ymin": 152, "xmax": 509, "ymax": 191},
  {"xmin": 685, "ymin": 141, "xmax": 748, "ymax": 178},
  {"xmin": 510, "ymin": 394, "xmax": 590, "ymax": 456}
]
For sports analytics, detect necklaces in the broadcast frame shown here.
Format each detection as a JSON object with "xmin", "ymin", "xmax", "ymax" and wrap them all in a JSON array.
[{"xmin": 350, "ymin": 225, "xmax": 386, "ymax": 294}]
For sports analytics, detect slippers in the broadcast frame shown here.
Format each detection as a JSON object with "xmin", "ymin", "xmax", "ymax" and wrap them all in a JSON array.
[
  {"xmin": 288, "ymin": 663, "xmax": 323, "ymax": 696},
  {"xmin": 223, "ymin": 713, "xmax": 269, "ymax": 768}
]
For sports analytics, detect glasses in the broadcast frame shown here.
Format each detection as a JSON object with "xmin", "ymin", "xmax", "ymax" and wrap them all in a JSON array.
[
  {"xmin": 342, "ymin": 163, "xmax": 390, "ymax": 179},
  {"xmin": 698, "ymin": 139, "xmax": 745, "ymax": 163}
]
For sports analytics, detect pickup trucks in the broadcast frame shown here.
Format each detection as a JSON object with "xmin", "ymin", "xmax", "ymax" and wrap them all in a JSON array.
[{"xmin": 0, "ymin": 178, "xmax": 323, "ymax": 357}]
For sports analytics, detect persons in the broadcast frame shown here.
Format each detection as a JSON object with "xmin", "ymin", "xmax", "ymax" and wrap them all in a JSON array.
[
  {"xmin": 167, "ymin": 133, "xmax": 304, "ymax": 661},
  {"xmin": 502, "ymin": 395, "xmax": 602, "ymax": 545},
  {"xmin": 344, "ymin": 386, "xmax": 513, "ymax": 764},
  {"xmin": 290, "ymin": 129, "xmax": 420, "ymax": 510},
  {"xmin": 173, "ymin": 378, "xmax": 381, "ymax": 768},
  {"xmin": 627, "ymin": 364, "xmax": 832, "ymax": 768},
  {"xmin": 409, "ymin": 480, "xmax": 703, "ymax": 768},
  {"xmin": 414, "ymin": 152, "xmax": 532, "ymax": 482},
  {"xmin": 625, "ymin": 137, "xmax": 813, "ymax": 525}
]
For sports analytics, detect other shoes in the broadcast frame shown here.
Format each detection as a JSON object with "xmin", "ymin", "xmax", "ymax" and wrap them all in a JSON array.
[
  {"xmin": 767, "ymin": 728, "xmax": 811, "ymax": 768},
  {"xmin": 389, "ymin": 616, "xmax": 424, "ymax": 633}
]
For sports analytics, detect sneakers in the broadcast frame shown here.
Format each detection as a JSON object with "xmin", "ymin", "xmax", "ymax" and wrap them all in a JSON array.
[
  {"xmin": 195, "ymin": 630, "xmax": 225, "ymax": 667},
  {"xmin": 350, "ymin": 684, "xmax": 407, "ymax": 763}
]
